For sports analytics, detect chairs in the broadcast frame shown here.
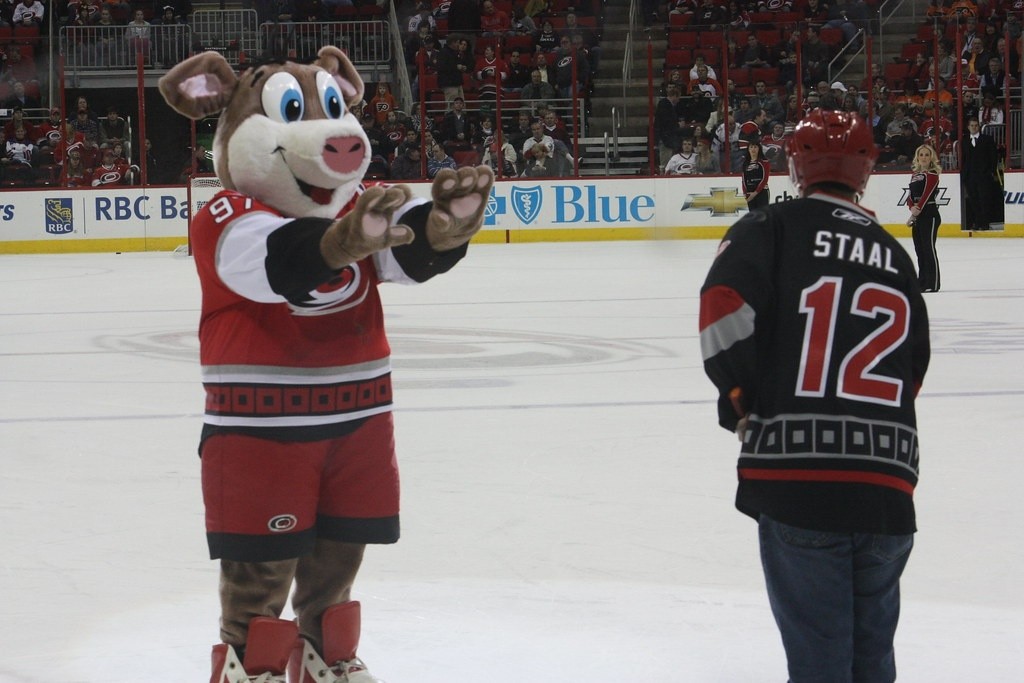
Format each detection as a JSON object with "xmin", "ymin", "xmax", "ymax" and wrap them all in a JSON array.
[{"xmin": 1, "ymin": 0, "xmax": 1024, "ymax": 191}]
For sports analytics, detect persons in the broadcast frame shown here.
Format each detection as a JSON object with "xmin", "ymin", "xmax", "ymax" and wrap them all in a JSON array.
[
  {"xmin": 368, "ymin": 81, "xmax": 400, "ymax": 126},
  {"xmin": 697, "ymin": 111, "xmax": 930, "ymax": 682},
  {"xmin": 694, "ymin": 139, "xmax": 721, "ymax": 174},
  {"xmin": 886, "ymin": 108, "xmax": 916, "ymax": 141},
  {"xmin": 980, "ymin": 58, "xmax": 1009, "ymax": 97},
  {"xmin": 688, "ymin": 54, "xmax": 717, "ymax": 81},
  {"xmin": 737, "ymin": 109, "xmax": 767, "ymax": 159},
  {"xmin": 438, "ymin": 97, "xmax": 472, "ymax": 150},
  {"xmin": 434, "ymin": 32, "xmax": 468, "ymax": 114},
  {"xmin": 664, "ymin": 137, "xmax": 697, "ymax": 175},
  {"xmin": 473, "ymin": 45, "xmax": 511, "ymax": 118},
  {"xmin": 97, "ymin": 106, "xmax": 131, "ymax": 157},
  {"xmin": 501, "ymin": 51, "xmax": 530, "ymax": 89},
  {"xmin": 0, "ymin": 0, "xmax": 1024, "ymax": 179},
  {"xmin": 519, "ymin": 70, "xmax": 558, "ymax": 114},
  {"xmin": 963, "ymin": 119, "xmax": 999, "ymax": 230},
  {"xmin": 739, "ymin": 141, "xmax": 770, "ymax": 212},
  {"xmin": 904, "ymin": 145, "xmax": 942, "ymax": 290},
  {"xmin": 748, "ymin": 80, "xmax": 785, "ymax": 133}
]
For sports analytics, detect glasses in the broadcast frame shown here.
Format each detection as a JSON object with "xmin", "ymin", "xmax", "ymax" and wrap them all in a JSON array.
[{"xmin": 459, "ymin": 43, "xmax": 467, "ymax": 46}]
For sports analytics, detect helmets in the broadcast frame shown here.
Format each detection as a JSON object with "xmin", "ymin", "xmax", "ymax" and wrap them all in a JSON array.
[
  {"xmin": 46, "ymin": 130, "xmax": 62, "ymax": 146},
  {"xmin": 163, "ymin": 6, "xmax": 175, "ymax": 12},
  {"xmin": 789, "ymin": 95, "xmax": 803, "ymax": 109},
  {"xmin": 783, "ymin": 107, "xmax": 878, "ymax": 205}
]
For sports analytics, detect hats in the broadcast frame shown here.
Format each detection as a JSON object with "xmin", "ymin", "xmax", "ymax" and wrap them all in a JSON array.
[
  {"xmin": 13, "ymin": 105, "xmax": 22, "ymax": 111},
  {"xmin": 104, "ymin": 149, "xmax": 114, "ymax": 157},
  {"xmin": 113, "ymin": 141, "xmax": 122, "ymax": 146},
  {"xmin": 807, "ymin": 92, "xmax": 820, "ymax": 102},
  {"xmin": 454, "ymin": 97, "xmax": 463, "ymax": 102},
  {"xmin": 51, "ymin": 106, "xmax": 60, "ymax": 113},
  {"xmin": 831, "ymin": 81, "xmax": 847, "ymax": 92}
]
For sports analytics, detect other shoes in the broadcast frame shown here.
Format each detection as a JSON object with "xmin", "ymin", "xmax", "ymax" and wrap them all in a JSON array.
[{"xmin": 209, "ymin": 601, "xmax": 381, "ymax": 683}]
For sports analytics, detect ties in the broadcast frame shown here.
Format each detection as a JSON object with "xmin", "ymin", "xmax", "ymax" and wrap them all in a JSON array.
[{"xmin": 971, "ymin": 138, "xmax": 975, "ymax": 147}]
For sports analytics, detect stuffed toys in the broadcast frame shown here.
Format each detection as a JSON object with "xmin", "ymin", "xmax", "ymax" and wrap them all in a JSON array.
[{"xmin": 155, "ymin": 46, "xmax": 493, "ymax": 683}]
